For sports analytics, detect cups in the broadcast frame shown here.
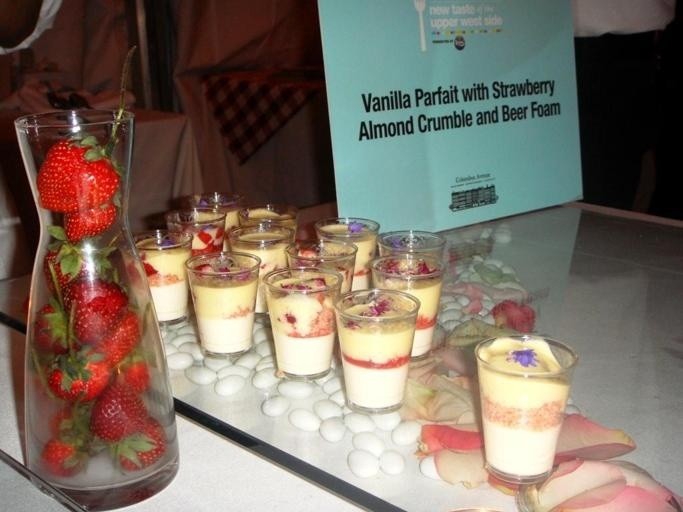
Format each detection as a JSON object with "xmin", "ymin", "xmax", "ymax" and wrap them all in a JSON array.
[
  {"xmin": 284, "ymin": 237, "xmax": 360, "ymax": 294},
  {"xmin": 315, "ymin": 215, "xmax": 380, "ymax": 290},
  {"xmin": 138, "ymin": 229, "xmax": 194, "ymax": 328},
  {"xmin": 367, "ymin": 253, "xmax": 445, "ymax": 362},
  {"xmin": 239, "ymin": 203, "xmax": 303, "ymax": 241},
  {"xmin": 333, "ymin": 287, "xmax": 421, "ymax": 412},
  {"xmin": 165, "ymin": 209, "xmax": 230, "ymax": 258},
  {"xmin": 473, "ymin": 334, "xmax": 579, "ymax": 486},
  {"xmin": 262, "ymin": 267, "xmax": 344, "ymax": 380},
  {"xmin": 184, "ymin": 251, "xmax": 262, "ymax": 358},
  {"xmin": 184, "ymin": 190, "xmax": 244, "ymax": 230},
  {"xmin": 227, "ymin": 223, "xmax": 297, "ymax": 325},
  {"xmin": 375, "ymin": 228, "xmax": 447, "ymax": 261}
]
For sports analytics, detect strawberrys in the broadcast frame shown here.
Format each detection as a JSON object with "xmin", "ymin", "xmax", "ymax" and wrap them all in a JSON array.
[
  {"xmin": 37, "ymin": 132, "xmax": 127, "ymax": 212},
  {"xmin": 115, "ymin": 360, "xmax": 148, "ymax": 395},
  {"xmin": 40, "ymin": 226, "xmax": 100, "ymax": 299},
  {"xmin": 62, "ymin": 204, "xmax": 116, "ymax": 243},
  {"xmin": 49, "ymin": 403, "xmax": 91, "ymax": 434},
  {"xmin": 106, "ymin": 417, "xmax": 168, "ymax": 471},
  {"xmin": 91, "ymin": 384, "xmax": 148, "ymax": 444},
  {"xmin": 41, "ymin": 430, "xmax": 88, "ymax": 478},
  {"xmin": 64, "ymin": 268, "xmax": 128, "ymax": 345},
  {"xmin": 42, "ymin": 345, "xmax": 112, "ymax": 406},
  {"xmin": 88, "ymin": 310, "xmax": 139, "ymax": 370},
  {"xmin": 33, "ymin": 296, "xmax": 79, "ymax": 355}
]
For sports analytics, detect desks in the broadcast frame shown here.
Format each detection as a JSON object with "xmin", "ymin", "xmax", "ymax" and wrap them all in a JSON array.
[{"xmin": 116, "ymin": 105, "xmax": 205, "ymax": 239}]
